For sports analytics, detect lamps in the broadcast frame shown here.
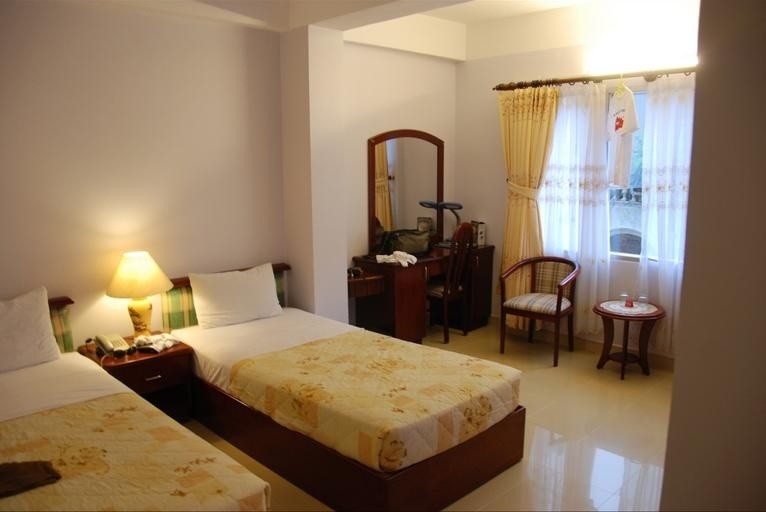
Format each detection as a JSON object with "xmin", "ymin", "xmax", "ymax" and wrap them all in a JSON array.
[
  {"xmin": 438, "ymin": 202, "xmax": 464, "ymax": 248},
  {"xmin": 105, "ymin": 250, "xmax": 175, "ymax": 336}
]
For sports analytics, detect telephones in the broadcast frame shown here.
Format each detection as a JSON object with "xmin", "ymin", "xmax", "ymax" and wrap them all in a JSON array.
[{"xmin": 94, "ymin": 334, "xmax": 131, "ymax": 358}]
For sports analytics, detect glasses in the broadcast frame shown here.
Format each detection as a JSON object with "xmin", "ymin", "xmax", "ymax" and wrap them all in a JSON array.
[{"xmin": 113, "ymin": 347, "xmax": 136, "ymax": 359}]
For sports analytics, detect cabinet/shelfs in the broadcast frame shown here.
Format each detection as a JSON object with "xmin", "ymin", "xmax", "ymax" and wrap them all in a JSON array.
[{"xmin": 352, "ymin": 240, "xmax": 495, "ymax": 344}]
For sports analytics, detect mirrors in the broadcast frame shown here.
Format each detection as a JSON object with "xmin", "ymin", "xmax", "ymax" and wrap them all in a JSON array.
[{"xmin": 367, "ymin": 128, "xmax": 445, "ymax": 244}]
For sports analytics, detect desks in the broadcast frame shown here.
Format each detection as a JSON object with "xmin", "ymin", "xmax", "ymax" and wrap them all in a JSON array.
[{"xmin": 592, "ymin": 298, "xmax": 667, "ymax": 380}]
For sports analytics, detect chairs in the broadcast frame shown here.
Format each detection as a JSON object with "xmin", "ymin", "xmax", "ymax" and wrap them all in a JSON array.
[
  {"xmin": 499, "ymin": 255, "xmax": 581, "ymax": 368},
  {"xmin": 427, "ymin": 245, "xmax": 480, "ymax": 344}
]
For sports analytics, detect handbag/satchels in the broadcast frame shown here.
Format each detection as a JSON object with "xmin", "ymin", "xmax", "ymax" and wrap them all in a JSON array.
[{"xmin": 378, "ymin": 230, "xmax": 430, "ymax": 256}]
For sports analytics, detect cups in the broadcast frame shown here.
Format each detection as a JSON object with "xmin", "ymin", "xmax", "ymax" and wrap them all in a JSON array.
[
  {"xmin": 619, "ymin": 293, "xmax": 628, "ymax": 307},
  {"xmin": 636, "ymin": 294, "xmax": 649, "ymax": 311}
]
